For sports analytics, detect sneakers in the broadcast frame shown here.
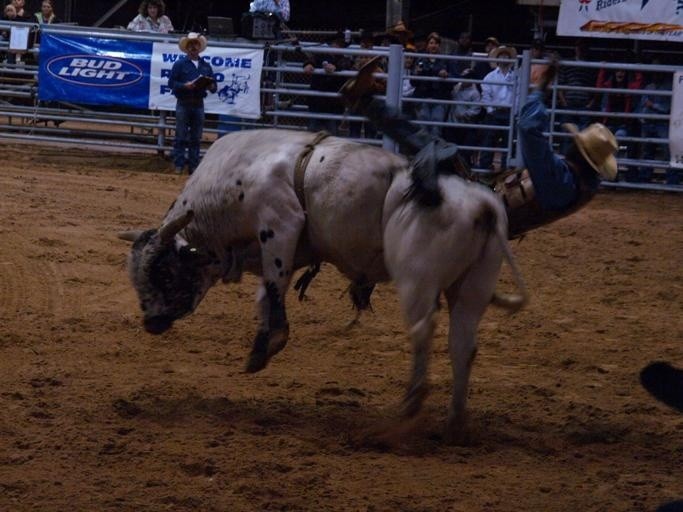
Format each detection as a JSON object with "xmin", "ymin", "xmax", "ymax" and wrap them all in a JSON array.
[
  {"xmin": 189, "ymin": 167, "xmax": 195, "ymax": 174},
  {"xmin": 175, "ymin": 166, "xmax": 182, "ymax": 174}
]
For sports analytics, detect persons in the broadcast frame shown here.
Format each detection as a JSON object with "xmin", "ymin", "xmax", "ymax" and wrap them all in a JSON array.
[
  {"xmin": 303, "ymin": 21, "xmax": 681, "ymax": 184},
  {"xmin": 249, "ymin": 0, "xmax": 291, "ymax": 21},
  {"xmin": 126, "ymin": 0, "xmax": 174, "ymax": 34},
  {"xmin": 336, "ymin": 53, "xmax": 618, "ymax": 241},
  {"xmin": 168, "ymin": 32, "xmax": 217, "ymax": 175},
  {"xmin": 0, "ymin": 0, "xmax": 62, "ymax": 71}
]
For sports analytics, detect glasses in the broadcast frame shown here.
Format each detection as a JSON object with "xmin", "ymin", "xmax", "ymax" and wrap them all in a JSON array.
[{"xmin": 333, "ymin": 38, "xmax": 345, "ymax": 45}]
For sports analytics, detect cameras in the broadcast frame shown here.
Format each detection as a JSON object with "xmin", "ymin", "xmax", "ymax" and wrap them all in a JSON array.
[{"xmin": 417, "ymin": 60, "xmax": 424, "ymax": 69}]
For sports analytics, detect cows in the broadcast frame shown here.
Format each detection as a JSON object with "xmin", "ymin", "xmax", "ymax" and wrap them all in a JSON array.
[{"xmin": 115, "ymin": 127, "xmax": 531, "ymax": 448}]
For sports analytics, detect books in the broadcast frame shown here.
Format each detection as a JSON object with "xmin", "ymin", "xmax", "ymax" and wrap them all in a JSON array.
[{"xmin": 193, "ymin": 75, "xmax": 214, "ymax": 87}]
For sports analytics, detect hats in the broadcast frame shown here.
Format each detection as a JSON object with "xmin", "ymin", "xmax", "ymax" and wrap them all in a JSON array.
[
  {"xmin": 486, "ymin": 37, "xmax": 499, "ymax": 45},
  {"xmin": 179, "ymin": 31, "xmax": 207, "ymax": 53},
  {"xmin": 458, "ymin": 68, "xmax": 475, "ymax": 79},
  {"xmin": 560, "ymin": 121, "xmax": 618, "ymax": 181},
  {"xmin": 488, "ymin": 45, "xmax": 517, "ymax": 69},
  {"xmin": 530, "ymin": 38, "xmax": 544, "ymax": 47},
  {"xmin": 391, "ymin": 21, "xmax": 414, "ymax": 39}
]
